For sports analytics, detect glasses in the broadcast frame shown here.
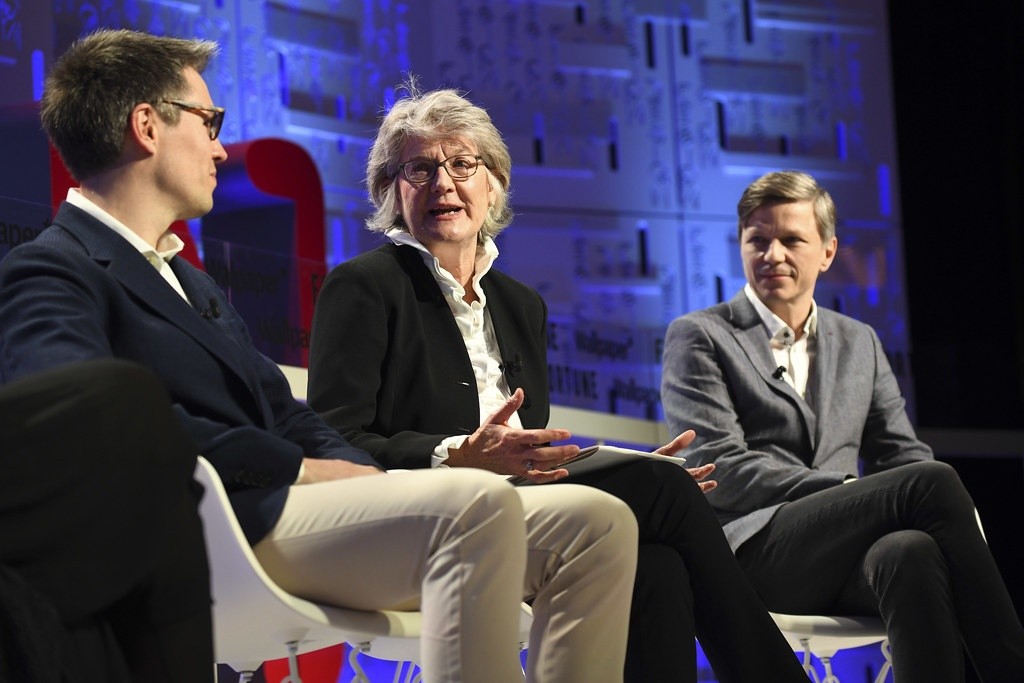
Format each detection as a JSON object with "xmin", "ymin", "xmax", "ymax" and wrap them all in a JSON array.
[
  {"xmin": 393, "ymin": 153, "xmax": 483, "ymax": 183},
  {"xmin": 152, "ymin": 96, "xmax": 227, "ymax": 141}
]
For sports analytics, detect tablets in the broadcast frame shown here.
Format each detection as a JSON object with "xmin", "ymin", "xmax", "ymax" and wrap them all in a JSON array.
[{"xmin": 505, "ymin": 444, "xmax": 686, "ymax": 485}]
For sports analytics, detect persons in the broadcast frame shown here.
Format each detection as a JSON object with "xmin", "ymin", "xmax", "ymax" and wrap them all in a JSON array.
[
  {"xmin": 1, "ymin": 361, "xmax": 217, "ymax": 679},
  {"xmin": 0, "ymin": 22, "xmax": 642, "ymax": 681},
  {"xmin": 311, "ymin": 85, "xmax": 813, "ymax": 683},
  {"xmin": 656, "ymin": 167, "xmax": 1024, "ymax": 683}
]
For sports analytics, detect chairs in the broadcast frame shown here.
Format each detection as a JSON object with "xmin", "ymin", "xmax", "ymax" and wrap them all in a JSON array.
[{"xmin": 193, "ymin": 458, "xmax": 545, "ymax": 683}]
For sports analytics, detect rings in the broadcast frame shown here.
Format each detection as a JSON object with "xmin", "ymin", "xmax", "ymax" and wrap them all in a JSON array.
[{"xmin": 524, "ymin": 458, "xmax": 533, "ymax": 471}]
